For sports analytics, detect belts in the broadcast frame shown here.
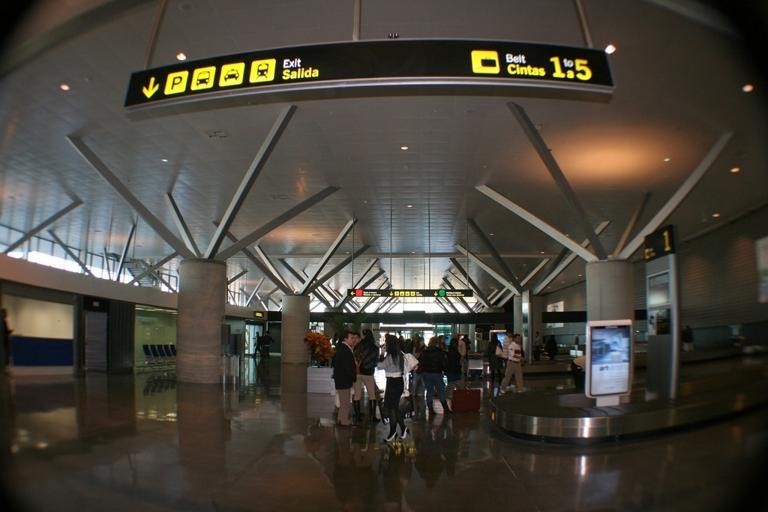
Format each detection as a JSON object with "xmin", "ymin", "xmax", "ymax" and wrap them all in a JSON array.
[{"xmin": 509, "ymin": 359, "xmax": 520, "ymax": 364}]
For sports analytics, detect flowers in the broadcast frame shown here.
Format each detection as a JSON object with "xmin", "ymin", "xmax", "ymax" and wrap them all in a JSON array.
[{"xmin": 307, "ymin": 330, "xmax": 337, "ymax": 367}]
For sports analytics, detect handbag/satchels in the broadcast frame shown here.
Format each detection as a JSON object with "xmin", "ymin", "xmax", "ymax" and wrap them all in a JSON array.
[{"xmin": 451, "ymin": 387, "xmax": 481, "ymax": 412}]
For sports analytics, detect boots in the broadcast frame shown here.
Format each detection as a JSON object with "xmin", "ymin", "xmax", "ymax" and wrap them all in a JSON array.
[
  {"xmin": 368, "ymin": 399, "xmax": 381, "ymax": 422},
  {"xmin": 439, "ymin": 400, "xmax": 455, "ymax": 417},
  {"xmin": 427, "ymin": 400, "xmax": 437, "ymax": 416},
  {"xmin": 352, "ymin": 399, "xmax": 365, "ymax": 423}
]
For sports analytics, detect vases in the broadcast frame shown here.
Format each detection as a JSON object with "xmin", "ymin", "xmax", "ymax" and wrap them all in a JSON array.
[{"xmin": 307, "ymin": 365, "xmax": 335, "ymax": 393}]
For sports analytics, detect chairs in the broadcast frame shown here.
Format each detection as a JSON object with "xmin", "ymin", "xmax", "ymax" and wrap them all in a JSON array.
[{"xmin": 141, "ymin": 344, "xmax": 177, "ymax": 367}]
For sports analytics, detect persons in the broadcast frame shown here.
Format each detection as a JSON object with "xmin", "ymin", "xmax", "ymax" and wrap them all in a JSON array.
[
  {"xmin": 328, "ymin": 425, "xmax": 419, "ymax": 512},
  {"xmin": 251, "ymin": 331, "xmax": 261, "ymax": 359},
  {"xmin": 684, "ymin": 325, "xmax": 695, "ymax": 352},
  {"xmin": 318, "ymin": 326, "xmax": 557, "ymax": 443},
  {"xmin": 0, "ymin": 307, "xmax": 15, "ymax": 378},
  {"xmin": 569, "ymin": 345, "xmax": 586, "ymax": 390},
  {"xmin": 258, "ymin": 330, "xmax": 276, "ymax": 361}
]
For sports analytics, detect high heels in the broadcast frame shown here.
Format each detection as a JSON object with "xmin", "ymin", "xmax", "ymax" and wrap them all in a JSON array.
[{"xmin": 384, "ymin": 426, "xmax": 411, "ymax": 443}]
